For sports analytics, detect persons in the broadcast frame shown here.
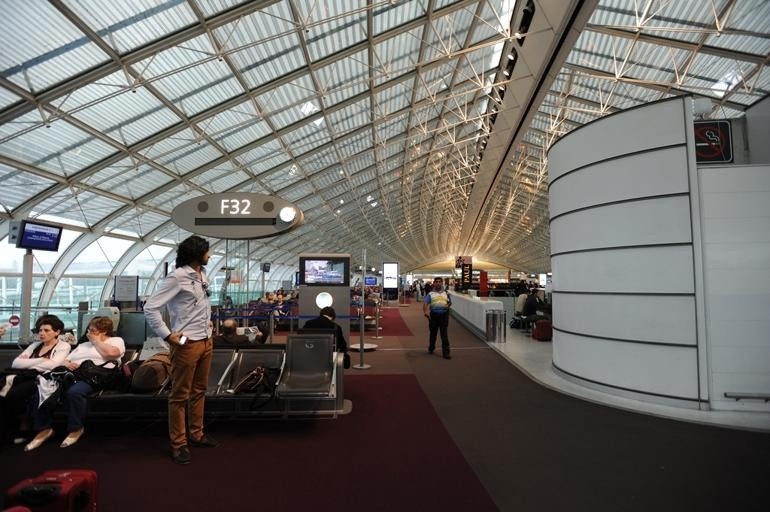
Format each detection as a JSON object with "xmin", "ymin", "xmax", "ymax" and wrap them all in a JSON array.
[
  {"xmin": 0, "ymin": 315, "xmax": 72, "ymax": 444},
  {"xmin": 349, "ymin": 274, "xmax": 383, "ymax": 321},
  {"xmin": 24, "ymin": 316, "xmax": 126, "ymax": 451},
  {"xmin": 143, "ymin": 236, "xmax": 220, "ymax": 464},
  {"xmin": 302, "ymin": 307, "xmax": 351, "ymax": 370},
  {"xmin": 211, "ymin": 287, "xmax": 300, "ymax": 349},
  {"xmin": 488, "ymin": 279, "xmax": 546, "ymax": 329},
  {"xmin": 423, "ymin": 277, "xmax": 452, "ymax": 360},
  {"xmin": 408, "ymin": 277, "xmax": 436, "ymax": 302}
]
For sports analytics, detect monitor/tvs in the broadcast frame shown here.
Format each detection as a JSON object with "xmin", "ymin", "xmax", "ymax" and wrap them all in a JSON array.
[
  {"xmin": 15, "ymin": 219, "xmax": 64, "ymax": 251},
  {"xmin": 365, "ymin": 277, "xmax": 377, "ymax": 285},
  {"xmin": 298, "ymin": 254, "xmax": 350, "ymax": 287}
]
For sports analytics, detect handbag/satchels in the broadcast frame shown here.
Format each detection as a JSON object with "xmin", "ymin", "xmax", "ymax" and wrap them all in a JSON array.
[
  {"xmin": 233, "ymin": 366, "xmax": 281, "ymax": 394},
  {"xmin": 75, "ymin": 352, "xmax": 174, "ymax": 392}
]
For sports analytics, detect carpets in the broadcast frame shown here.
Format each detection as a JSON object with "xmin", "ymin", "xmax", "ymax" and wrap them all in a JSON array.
[
  {"xmin": 287, "ymin": 308, "xmax": 414, "ymax": 336},
  {"xmin": 1, "ymin": 373, "xmax": 498, "ymax": 512}
]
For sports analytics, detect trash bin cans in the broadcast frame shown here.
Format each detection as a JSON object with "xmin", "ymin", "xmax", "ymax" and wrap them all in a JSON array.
[{"xmin": 486, "ymin": 309, "xmax": 506, "ymax": 343}]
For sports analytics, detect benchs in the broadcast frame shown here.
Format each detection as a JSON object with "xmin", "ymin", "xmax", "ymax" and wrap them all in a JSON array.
[{"xmin": 1, "ymin": 340, "xmax": 351, "ymax": 420}]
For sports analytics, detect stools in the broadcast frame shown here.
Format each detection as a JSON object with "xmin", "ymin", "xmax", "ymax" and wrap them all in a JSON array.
[
  {"xmin": 515, "ymin": 313, "xmax": 524, "ymax": 329},
  {"xmin": 527, "ymin": 319, "xmax": 538, "ymax": 337}
]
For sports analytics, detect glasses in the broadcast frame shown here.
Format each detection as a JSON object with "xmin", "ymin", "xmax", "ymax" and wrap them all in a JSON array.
[{"xmin": 202, "ymin": 282, "xmax": 212, "ymax": 297}]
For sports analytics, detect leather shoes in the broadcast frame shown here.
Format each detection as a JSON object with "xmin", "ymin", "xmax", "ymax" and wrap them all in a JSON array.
[
  {"xmin": 59, "ymin": 426, "xmax": 85, "ymax": 449},
  {"xmin": 189, "ymin": 433, "xmax": 218, "ymax": 447},
  {"xmin": 172, "ymin": 445, "xmax": 191, "ymax": 465},
  {"xmin": 24, "ymin": 429, "xmax": 54, "ymax": 451}
]
